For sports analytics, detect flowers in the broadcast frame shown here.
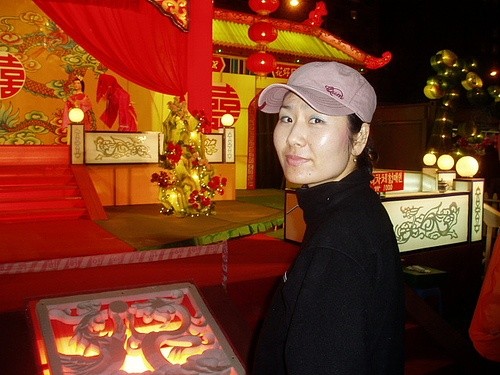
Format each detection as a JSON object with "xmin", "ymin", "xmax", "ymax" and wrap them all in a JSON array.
[{"xmin": 149, "ymin": 108, "xmax": 227, "ymax": 216}]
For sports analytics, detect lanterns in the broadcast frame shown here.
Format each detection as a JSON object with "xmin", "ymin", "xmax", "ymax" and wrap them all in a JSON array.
[
  {"xmin": 245, "ymin": 51, "xmax": 276, "ymax": 78},
  {"xmin": 247, "ymin": 20, "xmax": 278, "ymax": 47},
  {"xmin": 248, "ymin": 0, "xmax": 281, "ymax": 15}
]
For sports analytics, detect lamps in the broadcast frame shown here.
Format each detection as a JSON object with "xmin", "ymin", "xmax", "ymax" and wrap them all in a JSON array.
[
  {"xmin": 437, "ymin": 154, "xmax": 454, "ymax": 171},
  {"xmin": 68, "ymin": 107, "xmax": 85, "ymax": 122},
  {"xmin": 456, "ymin": 156, "xmax": 479, "ymax": 177},
  {"xmin": 220, "ymin": 113, "xmax": 235, "ymax": 127},
  {"xmin": 422, "ymin": 153, "xmax": 436, "ymax": 167}
]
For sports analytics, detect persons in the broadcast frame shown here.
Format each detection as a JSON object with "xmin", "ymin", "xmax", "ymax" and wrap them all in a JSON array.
[
  {"xmin": 248, "ymin": 61, "xmax": 407, "ymax": 374},
  {"xmin": 64, "ymin": 76, "xmax": 96, "ymax": 132},
  {"xmin": 467, "ymin": 232, "xmax": 500, "ymax": 375}
]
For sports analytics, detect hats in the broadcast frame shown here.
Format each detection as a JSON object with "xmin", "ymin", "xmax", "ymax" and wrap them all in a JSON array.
[{"xmin": 257, "ymin": 61, "xmax": 377, "ymax": 123}]
